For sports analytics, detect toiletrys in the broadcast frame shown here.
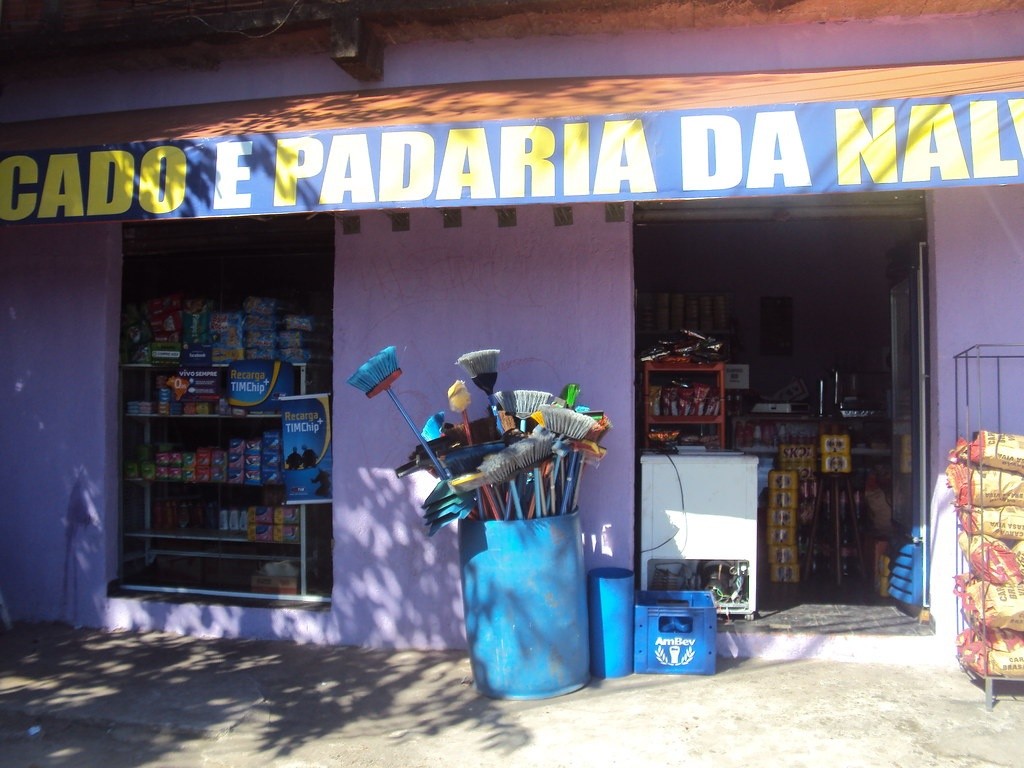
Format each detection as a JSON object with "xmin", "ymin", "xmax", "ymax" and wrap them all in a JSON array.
[{"xmin": 152, "ymin": 493, "xmax": 247, "ymax": 531}]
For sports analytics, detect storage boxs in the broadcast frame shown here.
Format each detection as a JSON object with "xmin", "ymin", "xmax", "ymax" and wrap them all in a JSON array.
[
  {"xmin": 633, "ymin": 589, "xmax": 718, "ymax": 676},
  {"xmin": 250, "ymin": 575, "xmax": 310, "ymax": 595},
  {"xmin": 128, "ymin": 295, "xmax": 330, "ymax": 546}
]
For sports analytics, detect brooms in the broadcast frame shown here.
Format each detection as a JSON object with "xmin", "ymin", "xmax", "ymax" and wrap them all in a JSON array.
[{"xmin": 345, "ymin": 342, "xmax": 615, "ymax": 520}]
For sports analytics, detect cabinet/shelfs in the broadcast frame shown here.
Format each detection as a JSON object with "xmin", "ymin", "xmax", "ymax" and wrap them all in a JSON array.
[
  {"xmin": 642, "ymin": 360, "xmax": 725, "ymax": 449},
  {"xmin": 121, "ymin": 360, "xmax": 333, "ymax": 601},
  {"xmin": 733, "ymin": 415, "xmax": 892, "ymax": 456}
]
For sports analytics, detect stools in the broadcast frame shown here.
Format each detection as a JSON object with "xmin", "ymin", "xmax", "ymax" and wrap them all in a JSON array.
[{"xmin": 803, "ymin": 470, "xmax": 867, "ymax": 589}]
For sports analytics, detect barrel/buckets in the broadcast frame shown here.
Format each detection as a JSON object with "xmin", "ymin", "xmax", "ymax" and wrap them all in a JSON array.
[
  {"xmin": 588, "ymin": 567, "xmax": 635, "ymax": 678},
  {"xmin": 459, "ymin": 505, "xmax": 591, "ymax": 701}
]
